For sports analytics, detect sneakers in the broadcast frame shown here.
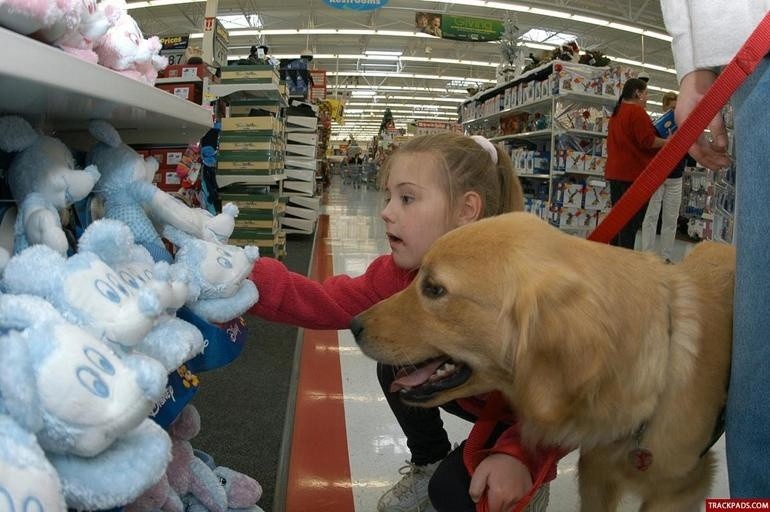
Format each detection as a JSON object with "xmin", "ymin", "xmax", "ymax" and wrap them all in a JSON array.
[{"xmin": 377, "ymin": 450, "xmax": 452, "ymax": 512}]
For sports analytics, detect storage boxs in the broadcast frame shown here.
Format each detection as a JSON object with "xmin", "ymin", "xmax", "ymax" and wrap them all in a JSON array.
[{"xmin": 138, "ymin": 61, "xmax": 288, "ymax": 261}]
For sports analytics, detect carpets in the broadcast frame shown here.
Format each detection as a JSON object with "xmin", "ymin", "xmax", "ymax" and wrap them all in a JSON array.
[{"xmin": 189, "ymin": 235, "xmax": 316, "ymax": 512}]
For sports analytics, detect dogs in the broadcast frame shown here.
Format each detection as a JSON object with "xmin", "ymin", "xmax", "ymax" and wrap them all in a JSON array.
[{"xmin": 348, "ymin": 210, "xmax": 737, "ymax": 512}]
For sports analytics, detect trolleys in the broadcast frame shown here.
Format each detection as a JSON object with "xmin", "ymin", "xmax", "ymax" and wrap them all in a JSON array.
[{"xmin": 338, "ymin": 160, "xmax": 382, "ymax": 191}]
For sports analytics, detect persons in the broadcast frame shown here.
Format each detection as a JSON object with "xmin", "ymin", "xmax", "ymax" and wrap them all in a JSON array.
[
  {"xmin": 424, "ymin": 15, "xmax": 442, "ymax": 37},
  {"xmin": 415, "ymin": 12, "xmax": 428, "ymax": 32},
  {"xmin": 659, "ymin": 0, "xmax": 770, "ymax": 499},
  {"xmin": 343, "ymin": 144, "xmax": 396, "ymax": 189},
  {"xmin": 0, "ymin": 0, "xmax": 265, "ymax": 512},
  {"xmin": 605, "ymin": 78, "xmax": 670, "ymax": 250},
  {"xmin": 246, "ymin": 132, "xmax": 557, "ymax": 512},
  {"xmin": 640, "ymin": 93, "xmax": 685, "ymax": 264}
]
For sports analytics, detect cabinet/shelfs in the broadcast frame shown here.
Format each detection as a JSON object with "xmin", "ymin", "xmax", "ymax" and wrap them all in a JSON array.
[{"xmin": 459, "ymin": 59, "xmax": 621, "ymax": 242}]
